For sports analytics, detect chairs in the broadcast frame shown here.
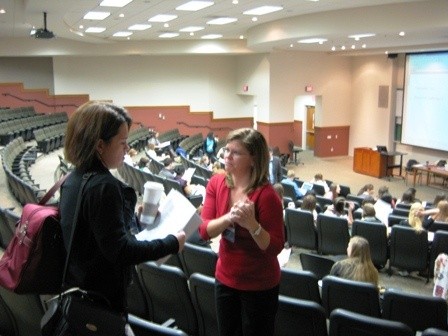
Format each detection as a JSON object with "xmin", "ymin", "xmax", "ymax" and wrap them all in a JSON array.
[{"xmin": 0, "ymin": 106, "xmax": 448, "ymax": 336}]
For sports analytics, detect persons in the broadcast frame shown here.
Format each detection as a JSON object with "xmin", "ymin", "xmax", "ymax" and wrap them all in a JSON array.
[
  {"xmin": 49, "ymin": 99, "xmax": 186, "ymax": 336},
  {"xmin": 124, "ymin": 128, "xmax": 448, "ymax": 272},
  {"xmin": 195, "ymin": 127, "xmax": 286, "ymax": 336},
  {"xmin": 318, "ymin": 236, "xmax": 379, "ymax": 299}
]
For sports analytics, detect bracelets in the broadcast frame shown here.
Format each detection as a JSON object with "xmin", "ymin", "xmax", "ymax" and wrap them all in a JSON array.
[{"xmin": 250, "ymin": 224, "xmax": 261, "ymax": 237}]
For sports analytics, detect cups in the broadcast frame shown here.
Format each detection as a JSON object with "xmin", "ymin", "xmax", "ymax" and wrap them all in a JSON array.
[{"xmin": 140, "ymin": 181, "xmax": 164, "ymax": 225}]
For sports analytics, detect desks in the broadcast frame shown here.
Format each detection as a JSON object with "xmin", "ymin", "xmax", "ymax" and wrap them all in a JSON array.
[
  {"xmin": 411, "ymin": 163, "xmax": 448, "ymax": 191},
  {"xmin": 379, "ymin": 153, "xmax": 408, "ymax": 182}
]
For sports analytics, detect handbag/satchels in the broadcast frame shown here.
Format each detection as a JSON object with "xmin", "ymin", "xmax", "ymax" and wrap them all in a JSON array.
[
  {"xmin": 0, "ymin": 173, "xmax": 71, "ymax": 294},
  {"xmin": 40, "ymin": 287, "xmax": 126, "ymax": 336}
]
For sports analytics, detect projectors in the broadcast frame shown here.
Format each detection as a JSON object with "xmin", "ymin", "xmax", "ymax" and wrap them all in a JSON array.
[{"xmin": 30, "ymin": 29, "xmax": 54, "ymax": 39}]
